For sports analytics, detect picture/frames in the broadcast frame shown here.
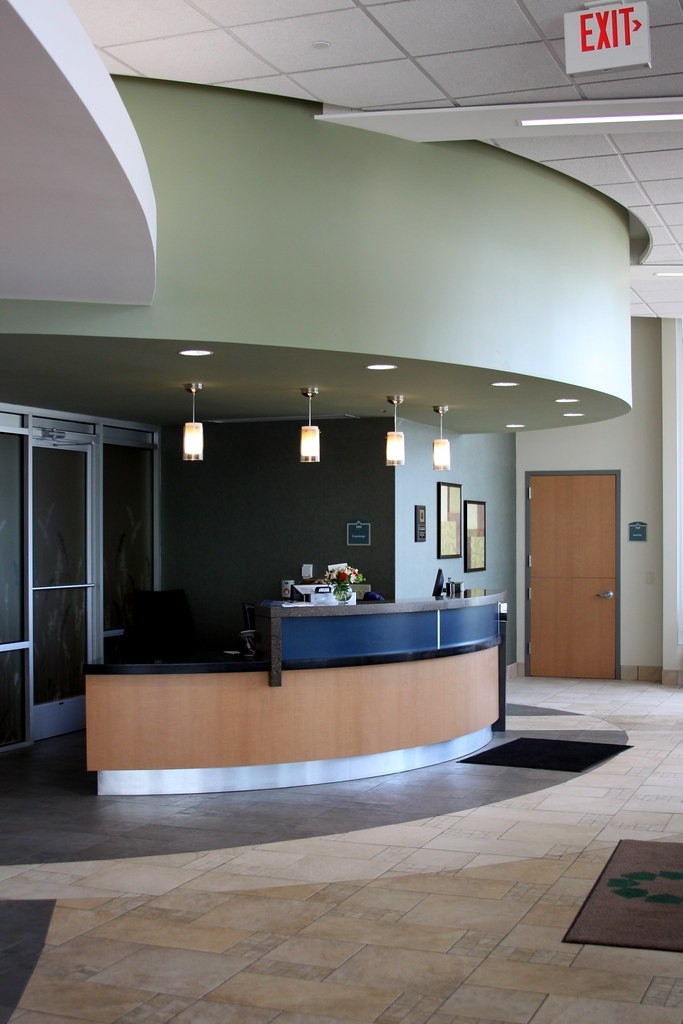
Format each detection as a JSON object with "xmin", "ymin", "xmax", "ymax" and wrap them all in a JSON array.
[
  {"xmin": 464, "ymin": 500, "xmax": 486, "ymax": 572},
  {"xmin": 437, "ymin": 481, "xmax": 463, "ymax": 558}
]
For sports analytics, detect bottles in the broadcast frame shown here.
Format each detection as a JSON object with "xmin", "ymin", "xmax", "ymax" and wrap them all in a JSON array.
[{"xmin": 446, "ymin": 577, "xmax": 451, "ymax": 598}]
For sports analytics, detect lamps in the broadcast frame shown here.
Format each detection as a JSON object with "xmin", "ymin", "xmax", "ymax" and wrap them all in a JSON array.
[
  {"xmin": 433, "ymin": 405, "xmax": 450, "ymax": 471},
  {"xmin": 183, "ymin": 381, "xmax": 204, "ymax": 461},
  {"xmin": 385, "ymin": 395, "xmax": 405, "ymax": 467},
  {"xmin": 301, "ymin": 387, "xmax": 320, "ymax": 463}
]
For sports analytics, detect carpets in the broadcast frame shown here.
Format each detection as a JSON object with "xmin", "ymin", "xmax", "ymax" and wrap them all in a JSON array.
[
  {"xmin": 561, "ymin": 839, "xmax": 683, "ymax": 953},
  {"xmin": 458, "ymin": 737, "xmax": 635, "ymax": 774}
]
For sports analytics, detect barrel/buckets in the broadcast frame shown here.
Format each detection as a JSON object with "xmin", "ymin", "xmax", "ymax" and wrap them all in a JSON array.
[{"xmin": 281, "ymin": 579, "xmax": 295, "ymax": 597}]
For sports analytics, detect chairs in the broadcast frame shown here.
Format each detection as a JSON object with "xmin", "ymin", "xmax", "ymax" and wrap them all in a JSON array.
[{"xmin": 124, "ymin": 588, "xmax": 201, "ymax": 645}]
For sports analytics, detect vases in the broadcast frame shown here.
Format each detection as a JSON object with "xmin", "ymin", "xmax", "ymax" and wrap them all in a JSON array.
[{"xmin": 334, "ymin": 584, "xmax": 352, "ymax": 604}]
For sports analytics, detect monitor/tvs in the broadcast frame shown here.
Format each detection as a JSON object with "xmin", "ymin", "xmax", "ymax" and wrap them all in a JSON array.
[
  {"xmin": 290, "ymin": 584, "xmax": 351, "ymax": 602},
  {"xmin": 432, "ymin": 569, "xmax": 444, "ymax": 596}
]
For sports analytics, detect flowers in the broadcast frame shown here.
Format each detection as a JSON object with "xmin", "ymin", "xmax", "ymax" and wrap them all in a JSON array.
[{"xmin": 325, "ymin": 566, "xmax": 366, "ymax": 595}]
